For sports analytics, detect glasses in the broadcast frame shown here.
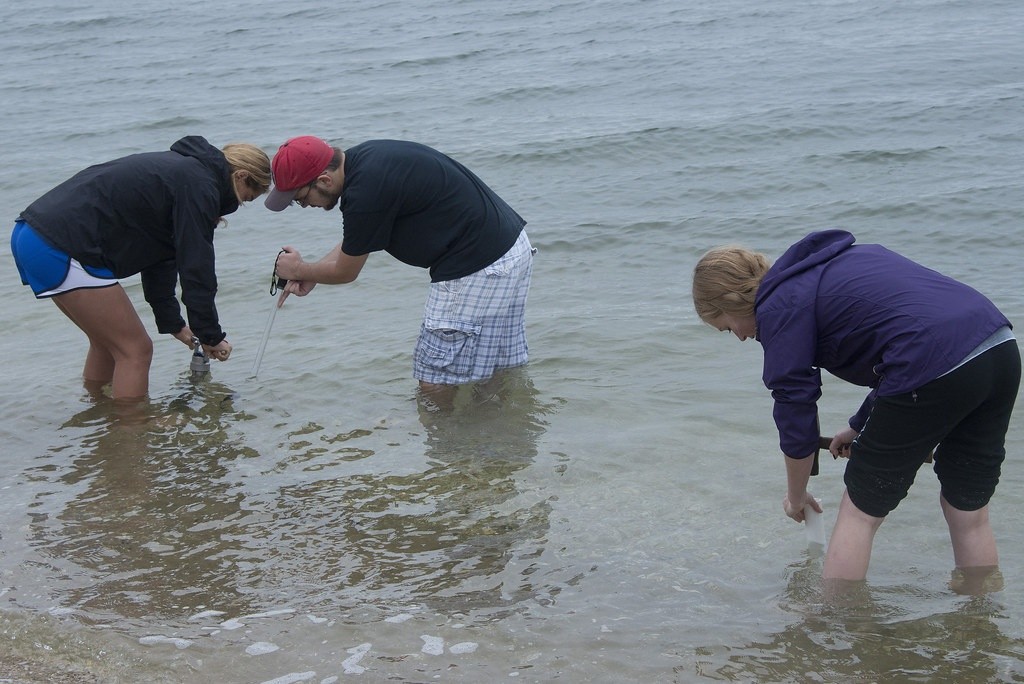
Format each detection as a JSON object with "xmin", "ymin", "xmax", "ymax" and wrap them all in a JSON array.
[{"xmin": 295, "ymin": 171, "xmax": 327, "ymax": 206}]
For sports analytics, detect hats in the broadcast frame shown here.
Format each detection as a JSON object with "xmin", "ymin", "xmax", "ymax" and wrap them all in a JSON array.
[{"xmin": 264, "ymin": 136, "xmax": 334, "ymax": 212}]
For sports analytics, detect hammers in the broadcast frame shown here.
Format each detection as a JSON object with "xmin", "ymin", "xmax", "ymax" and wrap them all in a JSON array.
[{"xmin": 809, "ymin": 436, "xmax": 932, "ymax": 477}]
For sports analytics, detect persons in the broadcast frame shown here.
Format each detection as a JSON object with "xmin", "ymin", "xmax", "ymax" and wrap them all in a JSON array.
[
  {"xmin": 264, "ymin": 135, "xmax": 538, "ymax": 387},
  {"xmin": 13, "ymin": 136, "xmax": 273, "ymax": 401},
  {"xmin": 691, "ymin": 229, "xmax": 1017, "ymax": 583}
]
